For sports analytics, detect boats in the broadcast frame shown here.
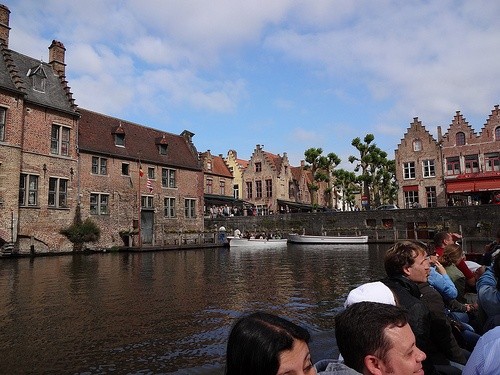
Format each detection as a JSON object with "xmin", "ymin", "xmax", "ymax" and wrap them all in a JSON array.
[{"xmin": 226, "ymin": 237, "xmax": 288, "ymax": 247}]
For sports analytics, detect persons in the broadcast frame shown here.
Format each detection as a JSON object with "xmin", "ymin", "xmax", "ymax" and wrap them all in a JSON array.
[
  {"xmin": 384, "ymin": 228, "xmax": 500, "ymax": 375},
  {"xmin": 313, "ymin": 301, "xmax": 427, "ymax": 374},
  {"xmin": 208, "ymin": 203, "xmax": 283, "ymax": 241},
  {"xmin": 337, "ymin": 280, "xmax": 400, "ymax": 361},
  {"xmin": 225, "ymin": 312, "xmax": 318, "ymax": 375}
]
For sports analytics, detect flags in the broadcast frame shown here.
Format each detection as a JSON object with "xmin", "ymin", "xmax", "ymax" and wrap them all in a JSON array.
[
  {"xmin": 146, "ymin": 178, "xmax": 154, "ymax": 193},
  {"xmin": 139, "ymin": 164, "xmax": 144, "ymax": 177}
]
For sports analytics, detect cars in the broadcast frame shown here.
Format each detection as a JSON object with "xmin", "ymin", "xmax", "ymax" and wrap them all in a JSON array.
[{"xmin": 374, "ymin": 204, "xmax": 400, "ymax": 210}]
